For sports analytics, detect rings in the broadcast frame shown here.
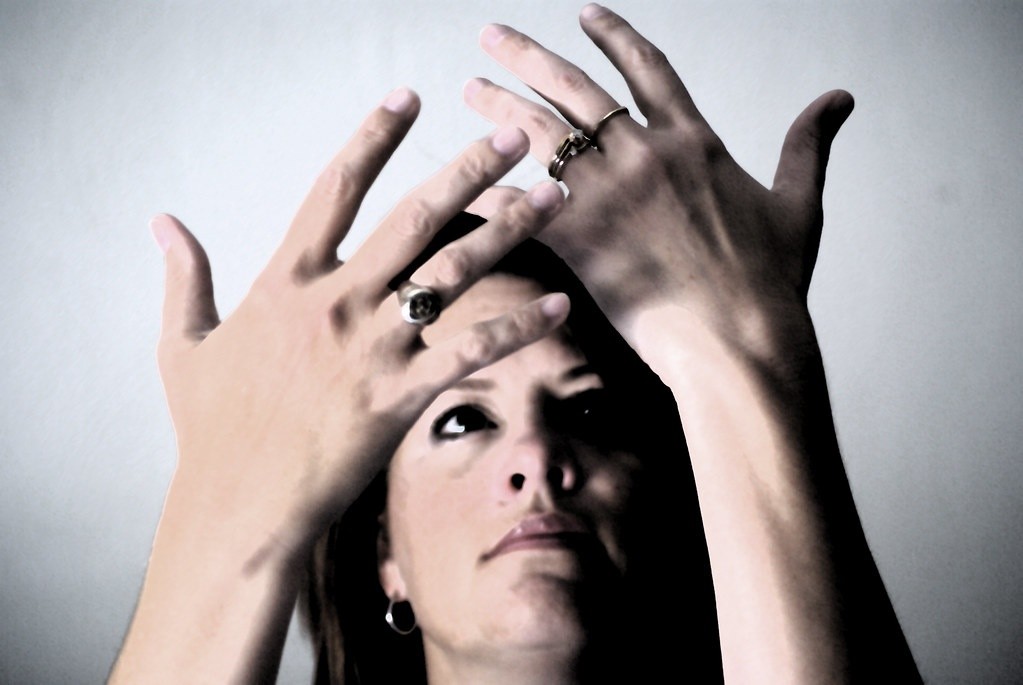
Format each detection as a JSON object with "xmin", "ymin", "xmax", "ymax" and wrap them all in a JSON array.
[
  {"xmin": 397, "ymin": 278, "xmax": 443, "ymax": 331},
  {"xmin": 586, "ymin": 103, "xmax": 632, "ymax": 147},
  {"xmin": 547, "ymin": 127, "xmax": 590, "ymax": 182}
]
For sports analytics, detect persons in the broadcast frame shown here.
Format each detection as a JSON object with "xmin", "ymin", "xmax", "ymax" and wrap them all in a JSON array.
[{"xmin": 122, "ymin": 1, "xmax": 939, "ymax": 685}]
